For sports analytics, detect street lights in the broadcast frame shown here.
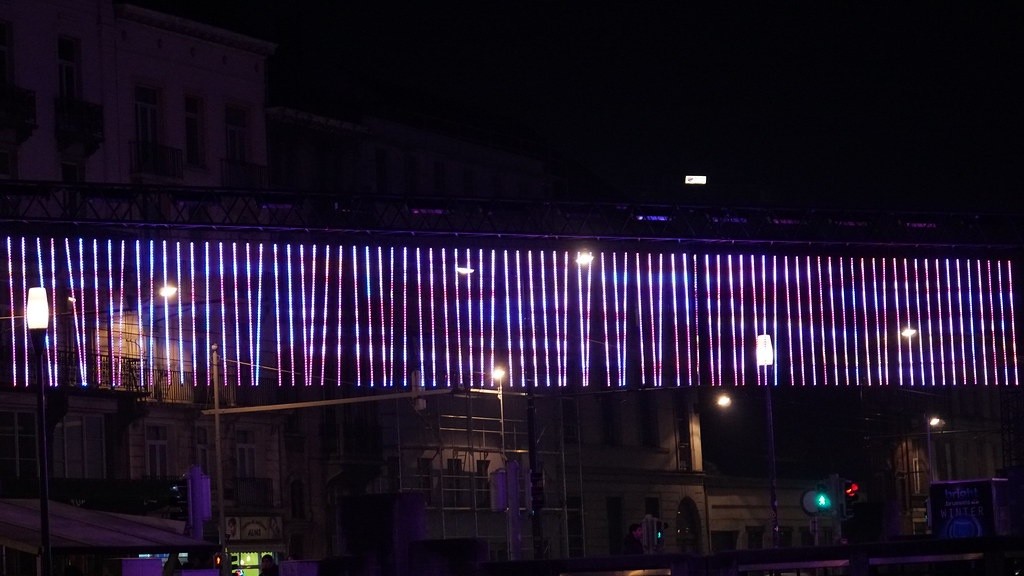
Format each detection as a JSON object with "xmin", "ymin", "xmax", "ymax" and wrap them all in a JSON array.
[
  {"xmin": 520, "ymin": 247, "xmax": 597, "ymax": 558},
  {"xmin": 700, "ymin": 393, "xmax": 733, "ymax": 551},
  {"xmin": 24, "ymin": 284, "xmax": 54, "ymax": 576},
  {"xmin": 927, "ymin": 405, "xmax": 941, "ymax": 482},
  {"xmin": 488, "ymin": 362, "xmax": 512, "ymax": 560},
  {"xmin": 859, "ymin": 327, "xmax": 917, "ymax": 540},
  {"xmin": 756, "ymin": 333, "xmax": 782, "ymax": 576}
]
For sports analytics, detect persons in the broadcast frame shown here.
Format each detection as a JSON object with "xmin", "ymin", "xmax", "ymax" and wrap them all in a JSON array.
[
  {"xmin": 257, "ymin": 554, "xmax": 280, "ymax": 576},
  {"xmin": 622, "ymin": 524, "xmax": 645, "ymax": 556},
  {"xmin": 163, "ymin": 547, "xmax": 182, "ymax": 576},
  {"xmin": 227, "ymin": 519, "xmax": 239, "ymax": 540}
]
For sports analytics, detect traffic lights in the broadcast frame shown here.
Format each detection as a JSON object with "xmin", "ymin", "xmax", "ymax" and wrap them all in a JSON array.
[
  {"xmin": 524, "ymin": 469, "xmax": 543, "ymax": 516},
  {"xmin": 844, "ymin": 481, "xmax": 859, "ymax": 518},
  {"xmin": 653, "ymin": 519, "xmax": 664, "ymax": 545},
  {"xmin": 478, "ymin": 473, "xmax": 506, "ymax": 512},
  {"xmin": 228, "ymin": 554, "xmax": 239, "ymax": 576},
  {"xmin": 215, "ymin": 555, "xmax": 221, "ymax": 567},
  {"xmin": 172, "ymin": 481, "xmax": 190, "ymax": 523},
  {"xmin": 817, "ymin": 482, "xmax": 833, "ymax": 516}
]
